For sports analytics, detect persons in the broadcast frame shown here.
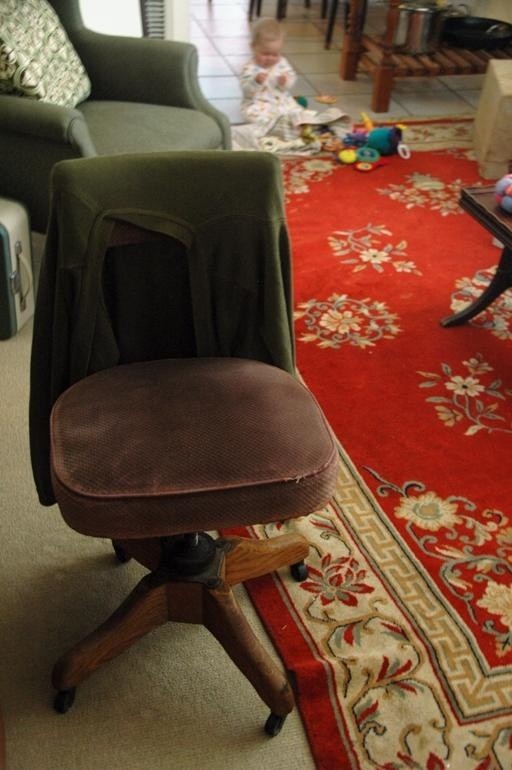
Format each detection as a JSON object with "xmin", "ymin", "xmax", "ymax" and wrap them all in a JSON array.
[{"xmin": 238, "ymin": 15, "xmax": 307, "ymax": 125}]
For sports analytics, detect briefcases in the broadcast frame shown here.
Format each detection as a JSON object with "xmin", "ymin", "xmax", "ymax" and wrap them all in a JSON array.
[{"xmin": 0, "ymin": 196, "xmax": 37, "ymax": 342}]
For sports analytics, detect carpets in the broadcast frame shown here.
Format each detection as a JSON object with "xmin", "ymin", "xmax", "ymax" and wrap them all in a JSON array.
[{"xmin": 217, "ymin": 114, "xmax": 512, "ymax": 769}]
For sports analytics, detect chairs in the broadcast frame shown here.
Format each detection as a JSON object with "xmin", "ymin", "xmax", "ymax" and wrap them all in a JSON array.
[
  {"xmin": 38, "ymin": 150, "xmax": 340, "ymax": 738},
  {"xmin": 0, "ymin": 0, "xmax": 231, "ymax": 236}
]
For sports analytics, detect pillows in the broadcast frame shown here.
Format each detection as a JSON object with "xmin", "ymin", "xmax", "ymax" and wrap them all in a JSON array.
[{"xmin": 0, "ymin": 1, "xmax": 93, "ymax": 111}]
